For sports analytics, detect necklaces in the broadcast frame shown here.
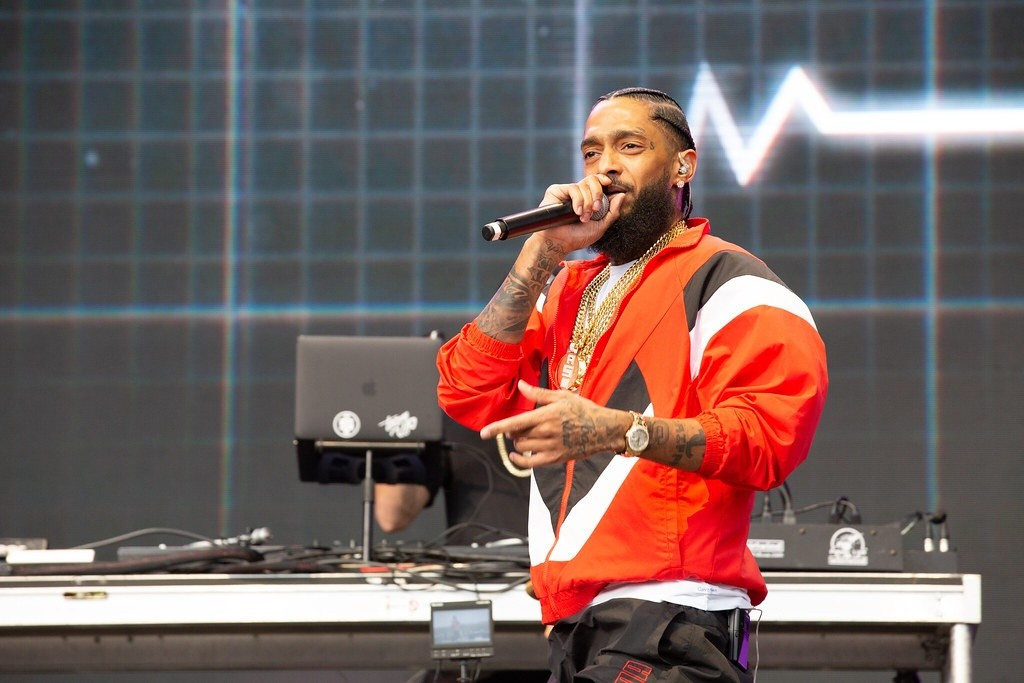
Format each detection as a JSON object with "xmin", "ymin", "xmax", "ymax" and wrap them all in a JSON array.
[{"xmin": 555, "ymin": 220, "xmax": 688, "ymax": 391}]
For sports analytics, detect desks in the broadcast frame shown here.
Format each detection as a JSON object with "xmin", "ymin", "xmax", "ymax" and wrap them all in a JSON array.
[{"xmin": 0, "ymin": 571, "xmax": 980, "ymax": 683}]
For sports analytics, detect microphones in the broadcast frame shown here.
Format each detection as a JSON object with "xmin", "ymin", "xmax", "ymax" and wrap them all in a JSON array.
[{"xmin": 482, "ymin": 192, "xmax": 610, "ymax": 242}]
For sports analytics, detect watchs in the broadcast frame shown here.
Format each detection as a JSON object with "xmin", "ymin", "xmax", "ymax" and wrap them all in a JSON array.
[{"xmin": 614, "ymin": 410, "xmax": 650, "ymax": 457}]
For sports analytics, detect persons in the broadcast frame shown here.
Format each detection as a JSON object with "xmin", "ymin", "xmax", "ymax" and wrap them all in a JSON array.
[
  {"xmin": 373, "ymin": 413, "xmax": 531, "ymax": 547},
  {"xmin": 437, "ymin": 88, "xmax": 828, "ymax": 683}
]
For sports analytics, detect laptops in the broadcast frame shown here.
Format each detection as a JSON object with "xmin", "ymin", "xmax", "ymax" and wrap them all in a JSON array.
[{"xmin": 293, "ymin": 336, "xmax": 444, "ymax": 441}]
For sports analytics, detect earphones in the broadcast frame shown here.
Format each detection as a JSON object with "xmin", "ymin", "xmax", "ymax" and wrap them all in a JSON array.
[{"xmin": 678, "ymin": 158, "xmax": 690, "ymax": 176}]
